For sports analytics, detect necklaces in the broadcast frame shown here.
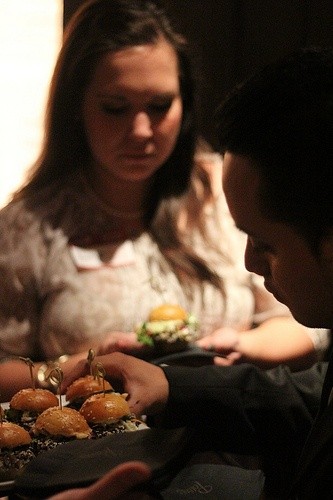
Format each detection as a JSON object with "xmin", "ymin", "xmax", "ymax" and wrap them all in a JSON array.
[{"xmin": 89, "ymin": 195, "xmax": 146, "ymax": 222}]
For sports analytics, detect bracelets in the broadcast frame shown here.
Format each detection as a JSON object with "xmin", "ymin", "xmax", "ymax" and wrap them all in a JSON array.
[{"xmin": 36, "ymin": 356, "xmax": 67, "ymax": 388}]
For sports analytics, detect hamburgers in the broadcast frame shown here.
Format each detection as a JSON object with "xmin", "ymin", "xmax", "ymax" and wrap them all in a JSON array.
[
  {"xmin": 135, "ymin": 304, "xmax": 204, "ymax": 347},
  {"xmin": 0, "ymin": 373, "xmax": 142, "ymax": 483}
]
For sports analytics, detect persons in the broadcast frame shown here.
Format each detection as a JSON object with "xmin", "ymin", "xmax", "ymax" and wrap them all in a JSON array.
[
  {"xmin": 43, "ymin": 52, "xmax": 333, "ymax": 500},
  {"xmin": 0, "ymin": 0, "xmax": 326, "ymax": 390}
]
